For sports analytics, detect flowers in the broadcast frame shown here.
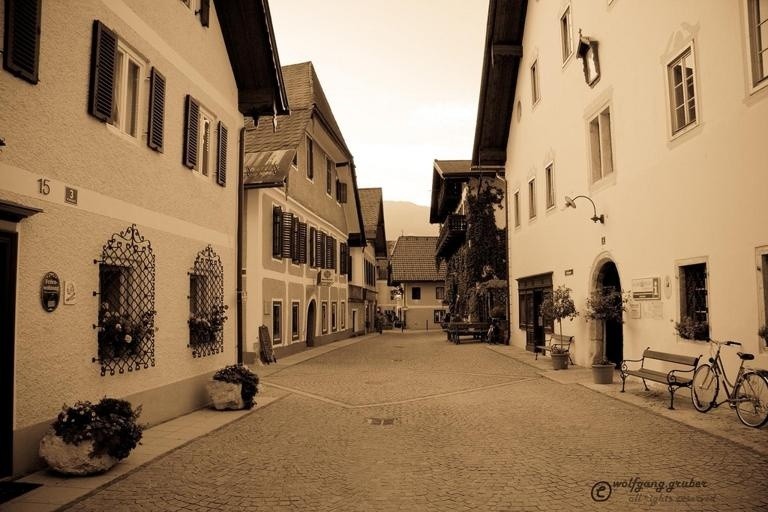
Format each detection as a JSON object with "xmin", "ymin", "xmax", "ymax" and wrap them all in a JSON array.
[
  {"xmin": 96, "ymin": 300, "xmax": 158, "ymax": 353},
  {"xmin": 212, "ymin": 363, "xmax": 259, "ymax": 409},
  {"xmin": 53, "ymin": 395, "xmax": 149, "ymax": 463},
  {"xmin": 188, "ymin": 302, "xmax": 230, "ymax": 336},
  {"xmin": 673, "ymin": 312, "xmax": 705, "ymax": 341}
]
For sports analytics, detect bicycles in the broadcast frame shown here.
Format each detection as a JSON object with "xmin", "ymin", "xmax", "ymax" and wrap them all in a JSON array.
[{"xmin": 691, "ymin": 336, "xmax": 768, "ymax": 428}]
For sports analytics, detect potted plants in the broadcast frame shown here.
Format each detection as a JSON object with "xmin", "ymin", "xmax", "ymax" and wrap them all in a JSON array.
[
  {"xmin": 539, "ymin": 283, "xmax": 580, "ymax": 370},
  {"xmin": 584, "ymin": 283, "xmax": 631, "ymax": 385}
]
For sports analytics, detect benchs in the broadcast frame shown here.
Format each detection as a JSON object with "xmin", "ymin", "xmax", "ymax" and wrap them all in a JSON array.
[
  {"xmin": 442, "ymin": 322, "xmax": 493, "ymax": 345},
  {"xmin": 618, "ymin": 345, "xmax": 704, "ymax": 411},
  {"xmin": 534, "ymin": 332, "xmax": 576, "ymax": 365}
]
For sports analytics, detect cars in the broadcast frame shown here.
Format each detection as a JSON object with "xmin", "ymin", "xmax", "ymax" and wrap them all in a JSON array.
[
  {"xmin": 376, "ymin": 311, "xmax": 402, "ymax": 330},
  {"xmin": 440, "ymin": 313, "xmax": 451, "ymax": 332}
]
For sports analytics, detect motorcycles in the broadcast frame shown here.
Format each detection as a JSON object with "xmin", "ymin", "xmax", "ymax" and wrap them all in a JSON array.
[{"xmin": 486, "ymin": 316, "xmax": 505, "ymax": 345}]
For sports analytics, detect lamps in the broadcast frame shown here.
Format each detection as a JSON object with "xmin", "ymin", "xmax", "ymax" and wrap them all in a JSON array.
[{"xmin": 563, "ymin": 193, "xmax": 607, "ymax": 224}]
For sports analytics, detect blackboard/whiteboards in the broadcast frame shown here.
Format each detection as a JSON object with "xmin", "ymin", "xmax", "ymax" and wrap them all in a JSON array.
[{"xmin": 259, "ymin": 324, "xmax": 274, "ymax": 362}]
